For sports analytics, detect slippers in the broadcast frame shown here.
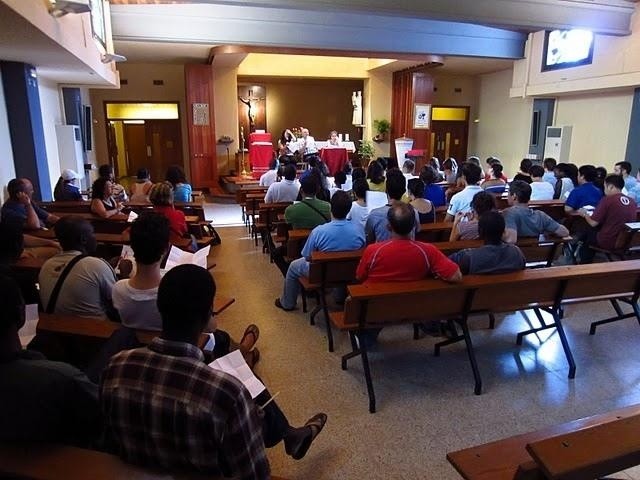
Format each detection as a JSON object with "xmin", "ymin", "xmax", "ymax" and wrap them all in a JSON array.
[
  {"xmin": 292, "ymin": 412, "xmax": 327, "ymax": 460},
  {"xmin": 238, "ymin": 324, "xmax": 259, "ymax": 369}
]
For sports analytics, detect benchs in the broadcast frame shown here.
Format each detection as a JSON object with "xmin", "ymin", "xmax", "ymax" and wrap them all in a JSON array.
[
  {"xmin": 1, "ymin": 175, "xmax": 238, "ymax": 478},
  {"xmin": 240, "ymin": 179, "xmax": 639, "ymax": 352},
  {"xmin": 441, "ymin": 403, "xmax": 638, "ymax": 478},
  {"xmin": 332, "ymin": 260, "xmax": 639, "ymax": 416}
]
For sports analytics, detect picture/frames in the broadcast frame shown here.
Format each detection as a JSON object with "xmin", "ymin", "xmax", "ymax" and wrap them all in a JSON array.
[
  {"xmin": 413, "ymin": 102, "xmax": 432, "ymax": 130},
  {"xmin": 89, "ymin": 0, "xmax": 107, "ymax": 51}
]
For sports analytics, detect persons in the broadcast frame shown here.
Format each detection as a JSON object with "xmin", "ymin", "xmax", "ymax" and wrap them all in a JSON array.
[
  {"xmin": 241, "ymin": 97, "xmax": 260, "ymax": 125},
  {"xmin": 0, "ymin": 276, "xmax": 98, "ymax": 447},
  {"xmin": 112, "ymin": 207, "xmax": 261, "ymax": 369},
  {"xmin": 38, "ymin": 214, "xmax": 132, "ymax": 321},
  {"xmin": 0, "ymin": 163, "xmax": 206, "ymax": 267},
  {"xmin": 278, "ymin": 128, "xmax": 321, "ymax": 167},
  {"xmin": 327, "ymin": 130, "xmax": 339, "ymax": 147},
  {"xmin": 99, "ymin": 265, "xmax": 328, "ymax": 480},
  {"xmin": 259, "ymin": 155, "xmax": 640, "ymax": 342}
]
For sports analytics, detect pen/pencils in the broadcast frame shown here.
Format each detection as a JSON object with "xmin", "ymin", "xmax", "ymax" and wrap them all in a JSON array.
[
  {"xmin": 123, "ymin": 251, "xmax": 128, "ymax": 258},
  {"xmin": 261, "ymin": 390, "xmax": 281, "ymax": 409}
]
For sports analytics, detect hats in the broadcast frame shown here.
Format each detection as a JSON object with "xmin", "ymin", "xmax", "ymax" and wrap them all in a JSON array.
[{"xmin": 61, "ymin": 169, "xmax": 82, "ymax": 180}]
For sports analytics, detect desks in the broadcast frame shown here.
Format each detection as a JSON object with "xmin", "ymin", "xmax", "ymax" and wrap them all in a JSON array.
[
  {"xmin": 277, "ymin": 139, "xmax": 357, "ymax": 154},
  {"xmin": 320, "ymin": 147, "xmax": 349, "ymax": 176}
]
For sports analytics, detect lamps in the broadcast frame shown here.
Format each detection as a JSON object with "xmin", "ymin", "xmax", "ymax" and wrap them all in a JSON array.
[
  {"xmin": 101, "ymin": 53, "xmax": 127, "ymax": 64},
  {"xmin": 47, "ymin": 0, "xmax": 94, "ymax": 18}
]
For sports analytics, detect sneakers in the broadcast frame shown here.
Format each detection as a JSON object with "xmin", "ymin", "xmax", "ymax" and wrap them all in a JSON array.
[
  {"xmin": 275, "ymin": 298, "xmax": 289, "ymax": 309},
  {"xmin": 420, "ymin": 322, "xmax": 441, "ymax": 334}
]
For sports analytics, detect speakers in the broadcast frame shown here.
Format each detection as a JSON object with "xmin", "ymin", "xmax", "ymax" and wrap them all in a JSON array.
[
  {"xmin": 532, "ymin": 111, "xmax": 539, "ymax": 146},
  {"xmin": 83, "ymin": 104, "xmax": 93, "ymax": 152}
]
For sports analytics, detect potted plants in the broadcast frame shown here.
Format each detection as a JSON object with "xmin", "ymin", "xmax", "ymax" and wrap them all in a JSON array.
[
  {"xmin": 373, "ymin": 118, "xmax": 393, "ymax": 141},
  {"xmin": 358, "ymin": 139, "xmax": 375, "ymax": 168}
]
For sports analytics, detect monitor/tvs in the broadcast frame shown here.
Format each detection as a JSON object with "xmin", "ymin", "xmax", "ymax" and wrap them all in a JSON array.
[{"xmin": 540, "ymin": 28, "xmax": 594, "ymax": 72}]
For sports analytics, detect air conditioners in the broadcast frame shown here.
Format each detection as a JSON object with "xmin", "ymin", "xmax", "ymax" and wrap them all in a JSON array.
[
  {"xmin": 543, "ymin": 126, "xmax": 572, "ymax": 163},
  {"xmin": 55, "ymin": 125, "xmax": 85, "ymax": 178}
]
